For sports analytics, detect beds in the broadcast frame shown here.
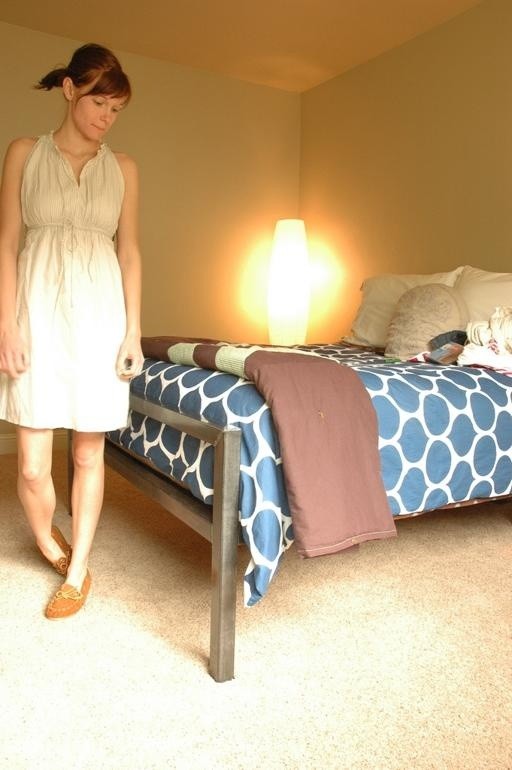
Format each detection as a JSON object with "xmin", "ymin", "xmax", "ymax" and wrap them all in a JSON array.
[{"xmin": 65, "ymin": 334, "xmax": 512, "ymax": 683}]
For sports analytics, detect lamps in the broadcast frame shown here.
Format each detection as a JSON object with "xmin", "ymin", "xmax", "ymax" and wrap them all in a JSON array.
[{"xmin": 267, "ymin": 218, "xmax": 309, "ymax": 345}]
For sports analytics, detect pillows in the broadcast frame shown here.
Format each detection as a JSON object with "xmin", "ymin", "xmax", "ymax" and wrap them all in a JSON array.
[
  {"xmin": 382, "ymin": 283, "xmax": 463, "ymax": 364},
  {"xmin": 339, "ymin": 266, "xmax": 463, "ymax": 352},
  {"xmin": 454, "ymin": 265, "xmax": 511, "ymax": 322}
]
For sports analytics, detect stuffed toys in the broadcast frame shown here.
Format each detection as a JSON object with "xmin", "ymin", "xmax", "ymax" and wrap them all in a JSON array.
[{"xmin": 457, "ymin": 306, "xmax": 512, "ymax": 378}]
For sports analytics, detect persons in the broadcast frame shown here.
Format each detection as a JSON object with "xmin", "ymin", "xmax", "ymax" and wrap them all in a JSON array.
[{"xmin": 0, "ymin": 44, "xmax": 145, "ymax": 618}]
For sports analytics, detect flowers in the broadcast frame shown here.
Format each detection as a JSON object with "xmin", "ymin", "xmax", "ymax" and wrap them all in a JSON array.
[{"xmin": 383, "ymin": 306, "xmax": 512, "ymax": 374}]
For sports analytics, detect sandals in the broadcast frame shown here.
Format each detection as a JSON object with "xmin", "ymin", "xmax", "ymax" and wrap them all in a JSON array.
[
  {"xmin": 47, "ymin": 524, "xmax": 73, "ymax": 577},
  {"xmin": 45, "ymin": 567, "xmax": 91, "ymax": 619}
]
